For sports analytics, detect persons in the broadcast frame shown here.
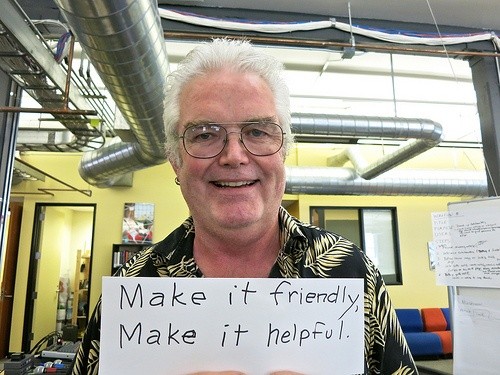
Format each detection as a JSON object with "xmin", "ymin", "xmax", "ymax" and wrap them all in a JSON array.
[{"xmin": 67, "ymin": 37, "xmax": 420, "ymax": 375}]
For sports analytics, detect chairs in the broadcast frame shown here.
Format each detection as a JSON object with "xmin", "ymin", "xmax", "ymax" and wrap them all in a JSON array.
[{"xmin": 401, "ymin": 304, "xmax": 453, "ymax": 360}]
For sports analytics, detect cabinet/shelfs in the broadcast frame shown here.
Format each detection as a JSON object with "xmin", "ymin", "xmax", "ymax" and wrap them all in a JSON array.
[
  {"xmin": 71, "ymin": 249, "xmax": 90, "ymax": 328},
  {"xmin": 112, "ymin": 244, "xmax": 151, "ymax": 277}
]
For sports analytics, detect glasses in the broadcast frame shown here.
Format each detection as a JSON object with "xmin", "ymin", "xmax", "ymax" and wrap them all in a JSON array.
[{"xmin": 179, "ymin": 121, "xmax": 286, "ymax": 159}]
